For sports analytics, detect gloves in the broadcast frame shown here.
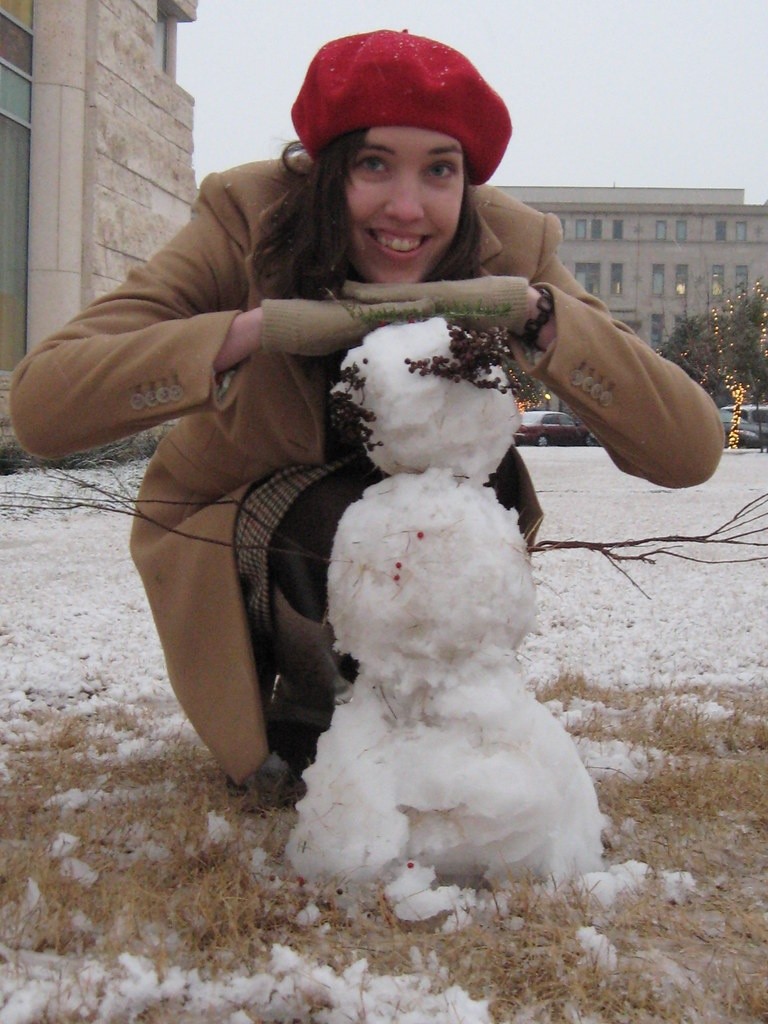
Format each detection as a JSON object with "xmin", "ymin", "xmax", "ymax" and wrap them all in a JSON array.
[
  {"xmin": 262, "ymin": 298, "xmax": 435, "ymax": 357},
  {"xmin": 342, "ymin": 275, "xmax": 528, "ymax": 336}
]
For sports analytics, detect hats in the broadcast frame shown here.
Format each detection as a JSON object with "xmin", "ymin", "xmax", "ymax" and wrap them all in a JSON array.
[{"xmin": 292, "ymin": 29, "xmax": 512, "ymax": 185}]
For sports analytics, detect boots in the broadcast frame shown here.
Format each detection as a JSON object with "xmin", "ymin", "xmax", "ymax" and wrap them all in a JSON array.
[{"xmin": 246, "ymin": 583, "xmax": 340, "ymax": 810}]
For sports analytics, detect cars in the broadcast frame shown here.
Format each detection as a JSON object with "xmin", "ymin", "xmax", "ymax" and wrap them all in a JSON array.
[
  {"xmin": 513, "ymin": 410, "xmax": 601, "ymax": 447},
  {"xmin": 718, "ymin": 405, "xmax": 768, "ymax": 448}
]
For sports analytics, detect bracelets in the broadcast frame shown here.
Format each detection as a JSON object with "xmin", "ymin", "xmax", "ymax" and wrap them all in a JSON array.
[{"xmin": 522, "ymin": 288, "xmax": 553, "ymax": 344}]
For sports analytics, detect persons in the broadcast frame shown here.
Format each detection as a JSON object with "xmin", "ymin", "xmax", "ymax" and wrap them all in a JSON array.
[{"xmin": 12, "ymin": 29, "xmax": 726, "ymax": 810}]
